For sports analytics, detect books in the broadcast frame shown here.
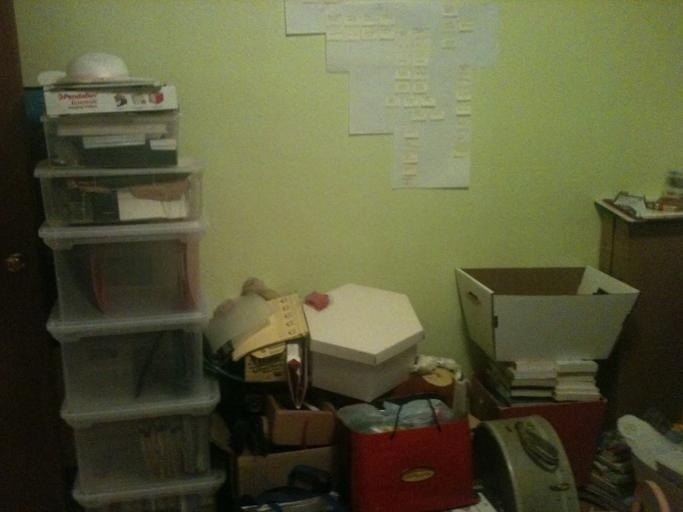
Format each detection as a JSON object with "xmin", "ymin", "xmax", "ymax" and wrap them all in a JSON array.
[
  {"xmin": 486, "ymin": 360, "xmax": 602, "ymax": 408},
  {"xmin": 580, "ymin": 450, "xmax": 634, "ymax": 510}
]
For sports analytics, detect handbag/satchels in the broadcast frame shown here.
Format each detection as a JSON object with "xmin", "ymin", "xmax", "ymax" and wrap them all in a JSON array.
[{"xmin": 351, "ymin": 396, "xmax": 482, "ymax": 512}]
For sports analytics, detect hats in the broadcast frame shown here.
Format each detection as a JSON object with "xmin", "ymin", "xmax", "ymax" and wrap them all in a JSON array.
[{"xmin": 54, "ymin": 53, "xmax": 146, "ymax": 85}]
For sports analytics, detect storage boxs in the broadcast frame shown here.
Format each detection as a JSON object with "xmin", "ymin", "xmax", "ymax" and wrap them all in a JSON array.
[
  {"xmin": 224, "ymin": 280, "xmax": 330, "ymax": 505},
  {"xmin": 331, "ymin": 369, "xmax": 477, "ymax": 512},
  {"xmin": 471, "ymin": 382, "xmax": 606, "ymax": 477},
  {"xmin": 34, "ymin": 378, "xmax": 224, "ymax": 511},
  {"xmin": 36, "ymin": 160, "xmax": 207, "ymax": 223},
  {"xmin": 36, "ymin": 219, "xmax": 211, "ymax": 324},
  {"xmin": 302, "ymin": 281, "xmax": 425, "ymax": 402},
  {"xmin": 453, "ymin": 264, "xmax": 641, "ymax": 361},
  {"xmin": 50, "ymin": 299, "xmax": 212, "ymax": 411},
  {"xmin": 38, "ymin": 110, "xmax": 181, "ymax": 168}
]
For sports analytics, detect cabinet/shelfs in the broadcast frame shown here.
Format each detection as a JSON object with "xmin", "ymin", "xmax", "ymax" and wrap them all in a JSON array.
[{"xmin": 593, "ymin": 197, "xmax": 682, "ymax": 434}]
